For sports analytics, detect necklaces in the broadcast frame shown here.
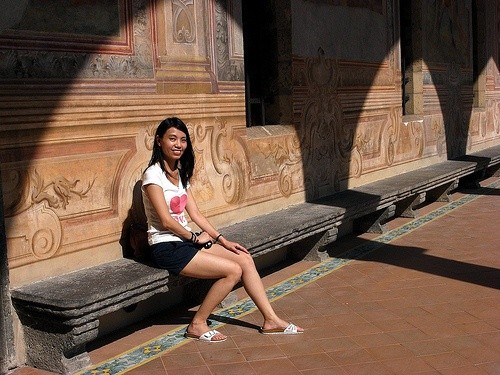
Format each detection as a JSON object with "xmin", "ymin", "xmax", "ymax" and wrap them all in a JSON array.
[{"xmin": 170, "ymin": 171, "xmax": 179, "ymax": 182}]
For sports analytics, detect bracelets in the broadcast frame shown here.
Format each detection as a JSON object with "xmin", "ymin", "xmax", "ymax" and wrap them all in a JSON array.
[
  {"xmin": 191, "ymin": 232, "xmax": 199, "ymax": 244},
  {"xmin": 216, "ymin": 234, "xmax": 224, "ymax": 242}
]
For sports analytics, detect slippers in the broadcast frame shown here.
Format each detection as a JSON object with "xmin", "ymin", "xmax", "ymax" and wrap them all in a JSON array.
[
  {"xmin": 184, "ymin": 330, "xmax": 227, "ymax": 343},
  {"xmin": 260, "ymin": 321, "xmax": 305, "ymax": 336}
]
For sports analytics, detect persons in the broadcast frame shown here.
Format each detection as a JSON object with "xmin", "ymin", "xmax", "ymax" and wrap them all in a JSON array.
[{"xmin": 141, "ymin": 117, "xmax": 306, "ymax": 344}]
